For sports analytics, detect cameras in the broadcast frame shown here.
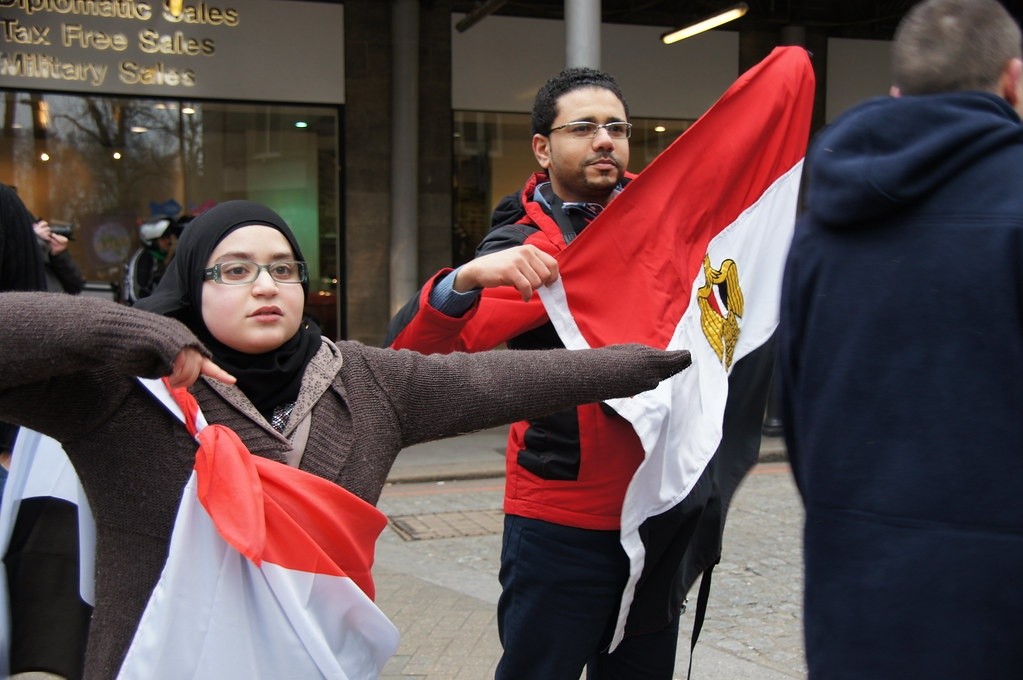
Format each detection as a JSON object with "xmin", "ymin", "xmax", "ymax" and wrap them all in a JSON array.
[{"xmin": 35, "ymin": 217, "xmax": 77, "ymax": 241}]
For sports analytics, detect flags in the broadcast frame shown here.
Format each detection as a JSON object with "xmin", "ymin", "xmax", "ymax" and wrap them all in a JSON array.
[{"xmin": 539, "ymin": 44, "xmax": 818, "ymax": 680}]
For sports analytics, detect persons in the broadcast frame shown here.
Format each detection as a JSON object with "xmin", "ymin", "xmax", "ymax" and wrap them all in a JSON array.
[
  {"xmin": 0, "ymin": 200, "xmax": 693, "ymax": 680},
  {"xmin": 776, "ymin": 0, "xmax": 1023, "ymax": 679},
  {"xmin": 0, "ymin": 185, "xmax": 185, "ymax": 307},
  {"xmin": 386, "ymin": 68, "xmax": 684, "ymax": 679}
]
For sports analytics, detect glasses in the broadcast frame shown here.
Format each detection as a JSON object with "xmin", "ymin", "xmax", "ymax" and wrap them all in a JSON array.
[
  {"xmin": 549, "ymin": 121, "xmax": 633, "ymax": 139},
  {"xmin": 203, "ymin": 260, "xmax": 308, "ymax": 285}
]
